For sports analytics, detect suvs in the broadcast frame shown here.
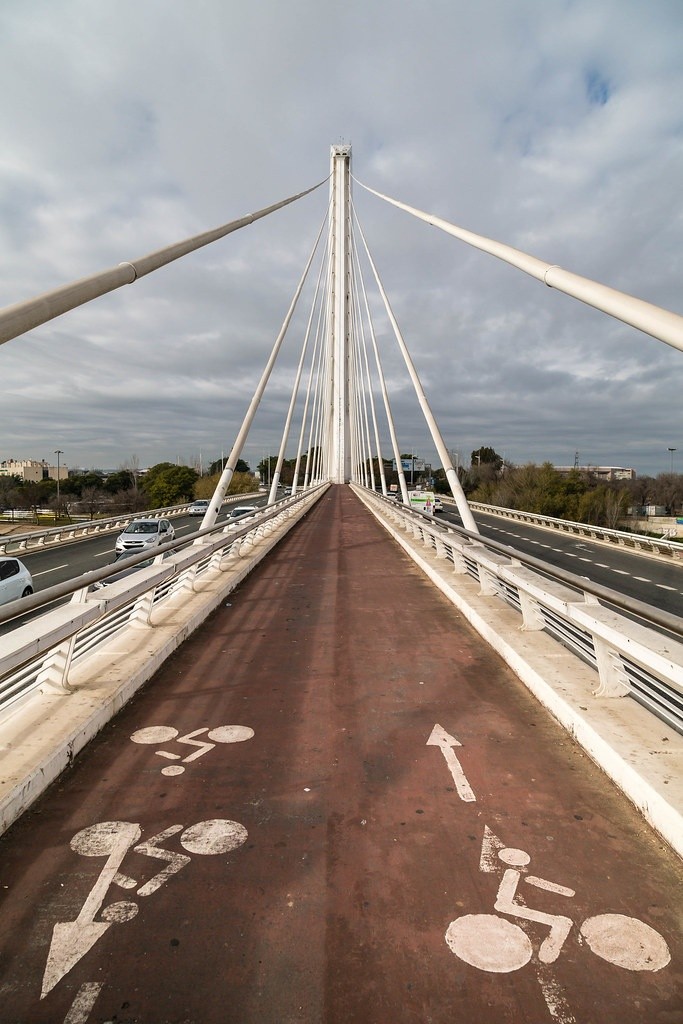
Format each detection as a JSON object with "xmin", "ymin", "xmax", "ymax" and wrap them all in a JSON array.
[{"xmin": 115, "ymin": 518, "xmax": 176, "ymax": 558}]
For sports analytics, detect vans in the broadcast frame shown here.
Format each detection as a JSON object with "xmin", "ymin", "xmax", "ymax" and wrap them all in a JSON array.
[{"xmin": 395, "ymin": 491, "xmax": 436, "ymax": 518}]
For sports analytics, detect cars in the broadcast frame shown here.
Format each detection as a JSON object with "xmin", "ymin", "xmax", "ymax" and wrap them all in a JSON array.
[
  {"xmin": 434, "ymin": 498, "xmax": 443, "ymax": 512},
  {"xmin": 277, "ymin": 482, "xmax": 282, "ymax": 488},
  {"xmin": 386, "ymin": 492, "xmax": 398, "ymax": 500},
  {"xmin": 0, "ymin": 556, "xmax": 35, "ymax": 607},
  {"xmin": 284, "ymin": 487, "xmax": 292, "ymax": 495},
  {"xmin": 188, "ymin": 500, "xmax": 211, "ymax": 517},
  {"xmin": 91, "ymin": 547, "xmax": 177, "ymax": 596},
  {"xmin": 223, "ymin": 506, "xmax": 260, "ymax": 533}
]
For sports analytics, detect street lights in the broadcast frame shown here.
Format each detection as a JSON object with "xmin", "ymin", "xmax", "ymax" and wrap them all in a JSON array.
[
  {"xmin": 53, "ymin": 449, "xmax": 64, "ymax": 518},
  {"xmin": 475, "ymin": 455, "xmax": 480, "ymax": 468},
  {"xmin": 453, "ymin": 452, "xmax": 459, "ymax": 474}
]
